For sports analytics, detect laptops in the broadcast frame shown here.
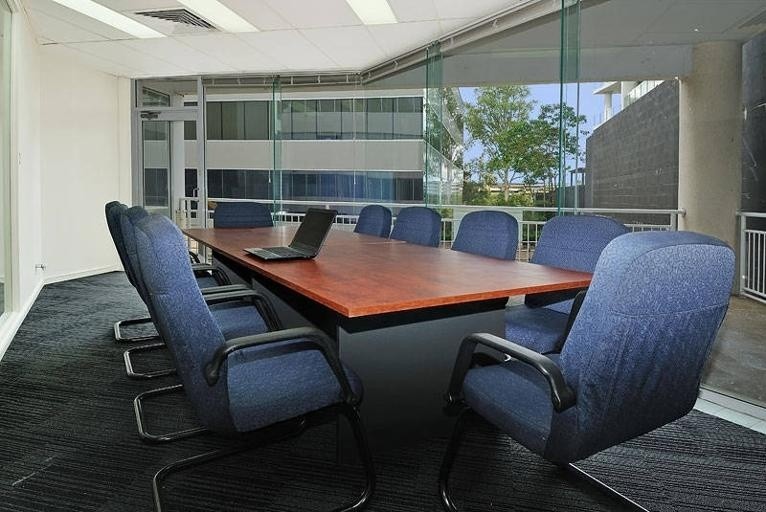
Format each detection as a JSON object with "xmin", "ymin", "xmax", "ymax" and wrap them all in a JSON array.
[{"xmin": 243, "ymin": 208, "xmax": 338, "ymax": 262}]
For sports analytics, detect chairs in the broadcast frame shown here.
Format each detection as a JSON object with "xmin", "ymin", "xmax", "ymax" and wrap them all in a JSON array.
[
  {"xmin": 118, "ymin": 208, "xmax": 324, "ymax": 444},
  {"xmin": 212, "ymin": 203, "xmax": 272, "ymax": 232},
  {"xmin": 131, "ymin": 217, "xmax": 371, "ymax": 512},
  {"xmin": 436, "ymin": 230, "xmax": 735, "ymax": 509},
  {"xmin": 101, "ymin": 202, "xmax": 231, "ymax": 344},
  {"xmin": 389, "ymin": 206, "xmax": 445, "ymax": 250},
  {"xmin": 453, "ymin": 210, "xmax": 518, "ymax": 260},
  {"xmin": 354, "ymin": 203, "xmax": 394, "ymax": 239},
  {"xmin": 109, "ymin": 206, "xmax": 243, "ymax": 381},
  {"xmin": 505, "ymin": 216, "xmax": 630, "ymax": 355}
]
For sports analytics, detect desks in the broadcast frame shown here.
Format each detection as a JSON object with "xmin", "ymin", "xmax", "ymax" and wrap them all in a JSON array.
[{"xmin": 182, "ymin": 222, "xmax": 595, "ymax": 463}]
